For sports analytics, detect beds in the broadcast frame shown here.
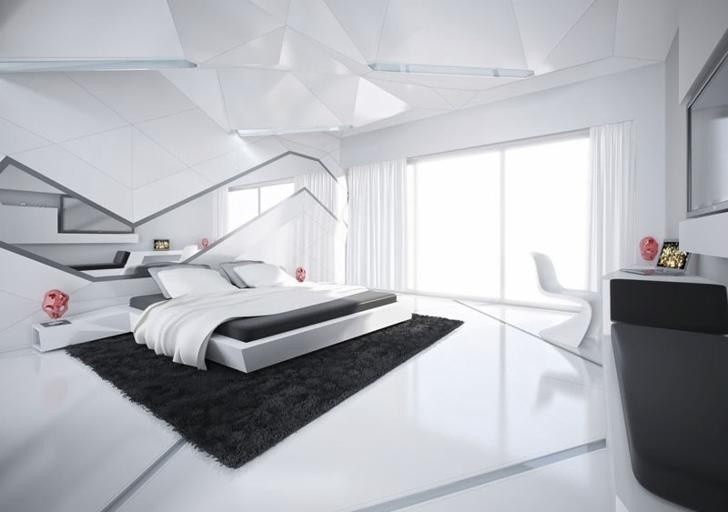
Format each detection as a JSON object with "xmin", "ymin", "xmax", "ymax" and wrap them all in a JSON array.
[{"xmin": 129, "ymin": 292, "xmax": 416, "ymax": 373}]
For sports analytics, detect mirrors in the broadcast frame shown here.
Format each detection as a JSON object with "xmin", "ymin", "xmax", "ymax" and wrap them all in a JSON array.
[{"xmin": 678, "ymin": 25, "xmax": 728, "ymax": 219}]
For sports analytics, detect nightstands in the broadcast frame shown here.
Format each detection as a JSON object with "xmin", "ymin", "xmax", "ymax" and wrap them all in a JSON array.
[{"xmin": 31, "ymin": 319, "xmax": 77, "ymax": 352}]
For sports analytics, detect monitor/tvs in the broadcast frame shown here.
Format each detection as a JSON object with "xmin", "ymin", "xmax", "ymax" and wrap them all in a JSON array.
[{"xmin": 684, "ymin": 46, "xmax": 728, "ymax": 219}]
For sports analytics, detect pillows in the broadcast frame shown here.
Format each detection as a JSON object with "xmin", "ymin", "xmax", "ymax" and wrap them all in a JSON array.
[
  {"xmin": 158, "ymin": 268, "xmax": 232, "ymax": 298},
  {"xmin": 235, "ymin": 263, "xmax": 298, "ymax": 288},
  {"xmin": 147, "ymin": 263, "xmax": 212, "ymax": 298},
  {"xmin": 220, "ymin": 260, "xmax": 266, "ymax": 289}
]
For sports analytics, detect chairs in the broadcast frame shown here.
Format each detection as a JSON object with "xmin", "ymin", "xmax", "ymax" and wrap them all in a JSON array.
[{"xmin": 528, "ymin": 250, "xmax": 602, "ymax": 351}]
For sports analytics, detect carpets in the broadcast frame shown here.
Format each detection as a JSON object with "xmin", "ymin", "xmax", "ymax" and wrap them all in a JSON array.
[{"xmin": 64, "ymin": 312, "xmax": 465, "ymax": 469}]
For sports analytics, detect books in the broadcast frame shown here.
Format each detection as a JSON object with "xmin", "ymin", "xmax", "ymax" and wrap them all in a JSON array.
[{"xmin": 620, "ymin": 265, "xmax": 687, "ymax": 276}]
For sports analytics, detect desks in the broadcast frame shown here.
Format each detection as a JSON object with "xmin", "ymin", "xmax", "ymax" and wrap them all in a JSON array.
[{"xmin": 600, "ymin": 266, "xmax": 727, "ymax": 512}]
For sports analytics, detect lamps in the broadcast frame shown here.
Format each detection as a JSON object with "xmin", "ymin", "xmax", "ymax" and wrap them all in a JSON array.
[
  {"xmin": 295, "ymin": 267, "xmax": 307, "ymax": 282},
  {"xmin": 43, "ymin": 288, "xmax": 69, "ymax": 326}
]
